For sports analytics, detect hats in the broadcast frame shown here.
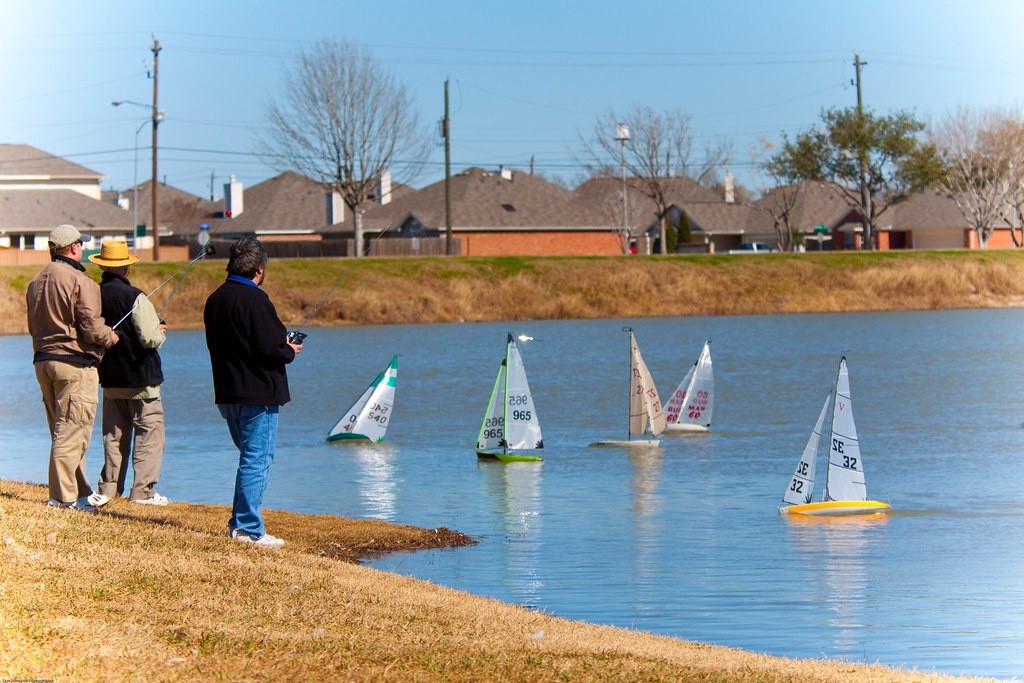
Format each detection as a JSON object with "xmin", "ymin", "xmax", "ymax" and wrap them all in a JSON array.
[
  {"xmin": 47, "ymin": 224, "xmax": 81, "ymax": 249},
  {"xmin": 87, "ymin": 240, "xmax": 141, "ymax": 267}
]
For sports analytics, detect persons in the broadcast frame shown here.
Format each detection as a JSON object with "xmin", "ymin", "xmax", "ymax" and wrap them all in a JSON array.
[
  {"xmin": 26, "ymin": 224, "xmax": 120, "ymax": 509},
  {"xmin": 204, "ymin": 237, "xmax": 302, "ymax": 545},
  {"xmin": 88, "ymin": 241, "xmax": 169, "ymax": 505}
]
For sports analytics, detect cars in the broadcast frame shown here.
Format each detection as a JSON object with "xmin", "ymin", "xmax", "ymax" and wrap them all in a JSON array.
[{"xmin": 727, "ymin": 239, "xmax": 781, "ymax": 255}]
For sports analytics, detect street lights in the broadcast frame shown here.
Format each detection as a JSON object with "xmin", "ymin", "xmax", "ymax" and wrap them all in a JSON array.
[
  {"xmin": 111, "ymin": 100, "xmax": 161, "ymax": 262},
  {"xmin": 132, "ymin": 114, "xmax": 164, "ymax": 256},
  {"xmin": 613, "ymin": 122, "xmax": 632, "ymax": 257}
]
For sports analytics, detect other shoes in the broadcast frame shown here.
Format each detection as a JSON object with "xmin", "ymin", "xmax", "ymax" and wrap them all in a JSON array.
[
  {"xmin": 77, "ymin": 491, "xmax": 110, "ymax": 507},
  {"xmin": 129, "ymin": 492, "xmax": 168, "ymax": 507},
  {"xmin": 228, "ymin": 528, "xmax": 284, "ymax": 546},
  {"xmin": 46, "ymin": 498, "xmax": 77, "ymax": 509}
]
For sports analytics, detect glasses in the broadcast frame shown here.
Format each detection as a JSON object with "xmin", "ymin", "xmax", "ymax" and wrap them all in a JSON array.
[{"xmin": 71, "ymin": 241, "xmax": 83, "ymax": 247}]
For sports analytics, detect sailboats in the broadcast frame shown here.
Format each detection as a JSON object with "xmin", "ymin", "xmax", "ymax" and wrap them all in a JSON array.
[
  {"xmin": 325, "ymin": 352, "xmax": 400, "ymax": 444},
  {"xmin": 476, "ymin": 332, "xmax": 545, "ymax": 464},
  {"xmin": 646, "ymin": 339, "xmax": 713, "ymax": 434},
  {"xmin": 589, "ymin": 325, "xmax": 668, "ymax": 446},
  {"xmin": 777, "ymin": 360, "xmax": 893, "ymax": 517}
]
